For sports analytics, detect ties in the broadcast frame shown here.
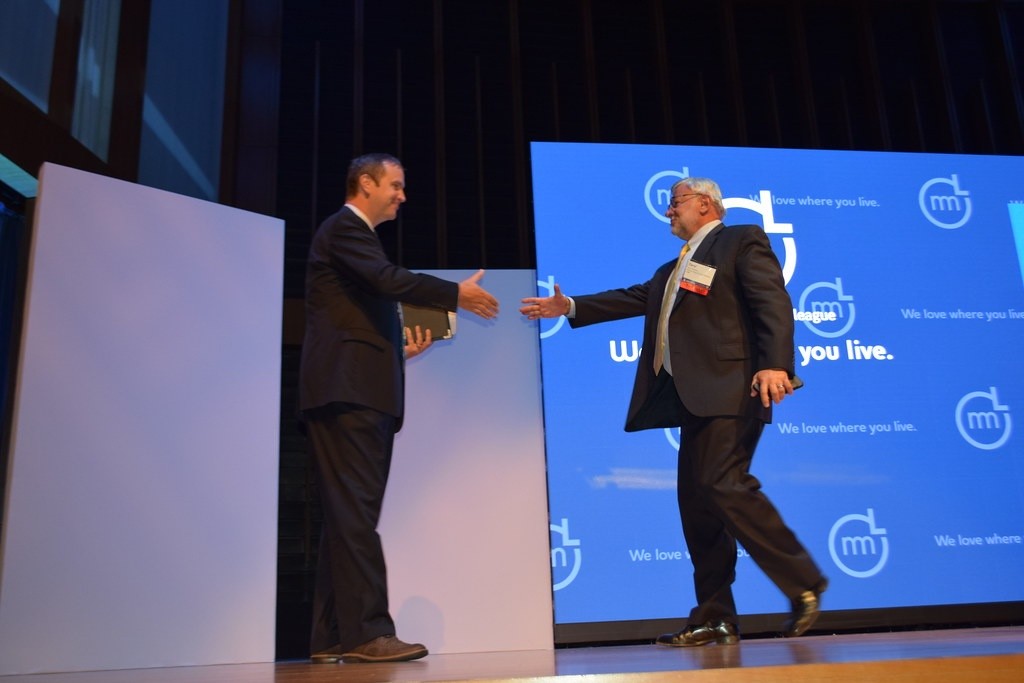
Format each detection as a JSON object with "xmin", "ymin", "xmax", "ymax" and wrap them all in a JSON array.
[{"xmin": 653, "ymin": 243, "xmax": 690, "ymax": 377}]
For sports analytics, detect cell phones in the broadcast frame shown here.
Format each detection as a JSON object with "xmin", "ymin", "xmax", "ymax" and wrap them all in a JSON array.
[{"xmin": 753, "ymin": 375, "xmax": 804, "ymax": 396}]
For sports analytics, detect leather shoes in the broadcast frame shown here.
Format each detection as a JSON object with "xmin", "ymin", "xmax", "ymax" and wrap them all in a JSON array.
[
  {"xmin": 788, "ymin": 578, "xmax": 828, "ymax": 637},
  {"xmin": 312, "ymin": 634, "xmax": 430, "ymax": 661},
  {"xmin": 655, "ymin": 617, "xmax": 740, "ymax": 645}
]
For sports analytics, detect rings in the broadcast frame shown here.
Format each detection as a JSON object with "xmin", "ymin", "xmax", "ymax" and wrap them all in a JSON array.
[
  {"xmin": 778, "ymin": 384, "xmax": 784, "ymax": 388},
  {"xmin": 416, "ymin": 343, "xmax": 422, "ymax": 347}
]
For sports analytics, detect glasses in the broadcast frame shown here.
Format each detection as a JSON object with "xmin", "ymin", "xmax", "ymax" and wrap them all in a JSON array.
[{"xmin": 667, "ymin": 193, "xmax": 704, "ymax": 208}]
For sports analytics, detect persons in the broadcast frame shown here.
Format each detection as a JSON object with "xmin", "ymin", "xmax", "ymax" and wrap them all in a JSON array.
[
  {"xmin": 519, "ymin": 177, "xmax": 831, "ymax": 648},
  {"xmin": 294, "ymin": 151, "xmax": 500, "ymax": 664}
]
